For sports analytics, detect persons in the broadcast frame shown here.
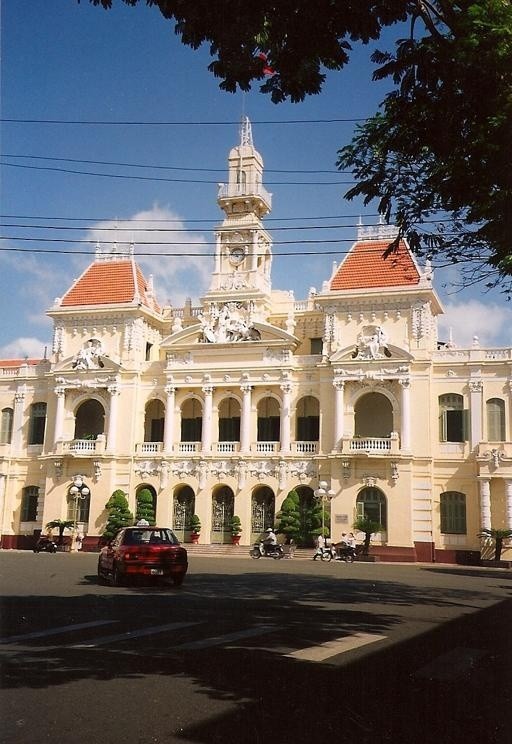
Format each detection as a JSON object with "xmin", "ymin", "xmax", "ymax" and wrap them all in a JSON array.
[
  {"xmin": 312, "ymin": 530, "xmax": 359, "ymax": 561},
  {"xmin": 38, "ymin": 527, "xmax": 53, "ymax": 550},
  {"xmin": 259, "ymin": 528, "xmax": 276, "ymax": 556}
]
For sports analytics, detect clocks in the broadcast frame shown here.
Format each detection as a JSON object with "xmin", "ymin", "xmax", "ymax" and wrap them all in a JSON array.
[{"xmin": 230, "ymin": 246, "xmax": 245, "ymax": 265}]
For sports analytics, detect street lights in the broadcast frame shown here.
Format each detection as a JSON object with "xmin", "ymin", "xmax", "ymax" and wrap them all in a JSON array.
[
  {"xmin": 68, "ymin": 477, "xmax": 90, "ymax": 553},
  {"xmin": 313, "ymin": 481, "xmax": 336, "ymax": 539}
]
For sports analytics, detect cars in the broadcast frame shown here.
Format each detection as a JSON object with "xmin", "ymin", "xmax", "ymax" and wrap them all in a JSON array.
[{"xmin": 96, "ymin": 518, "xmax": 189, "ymax": 585}]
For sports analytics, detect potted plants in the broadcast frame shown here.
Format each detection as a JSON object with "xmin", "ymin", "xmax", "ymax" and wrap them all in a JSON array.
[
  {"xmin": 230, "ymin": 516, "xmax": 241, "ymax": 544},
  {"xmin": 190, "ymin": 514, "xmax": 200, "ymax": 544}
]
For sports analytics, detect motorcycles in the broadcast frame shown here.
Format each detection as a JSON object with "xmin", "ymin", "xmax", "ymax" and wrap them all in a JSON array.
[
  {"xmin": 250, "ymin": 540, "xmax": 285, "ymax": 559},
  {"xmin": 313, "ymin": 544, "xmax": 357, "ymax": 563},
  {"xmin": 33, "ymin": 535, "xmax": 58, "ymax": 553}
]
